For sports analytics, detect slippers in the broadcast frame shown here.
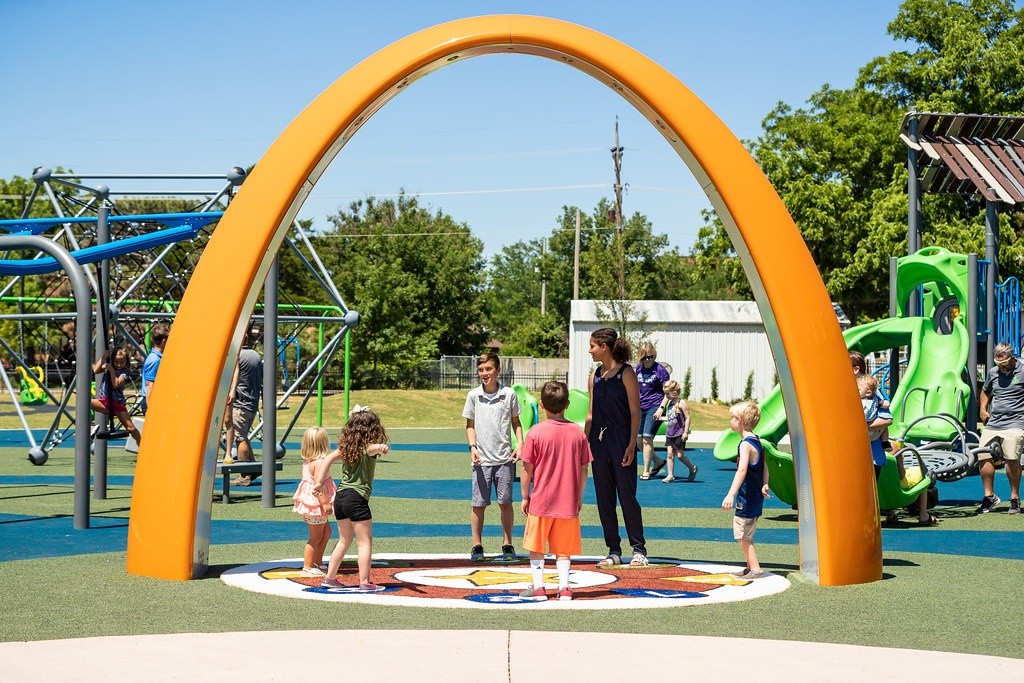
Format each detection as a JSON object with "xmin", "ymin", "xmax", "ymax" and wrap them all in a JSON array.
[
  {"xmin": 917, "ymin": 514, "xmax": 939, "ymax": 524},
  {"xmin": 650, "ymin": 460, "xmax": 667, "ymax": 477},
  {"xmin": 596, "ymin": 554, "xmax": 624, "ymax": 567},
  {"xmin": 640, "ymin": 475, "xmax": 651, "ymax": 480},
  {"xmin": 629, "ymin": 554, "xmax": 649, "ymax": 567},
  {"xmin": 885, "ymin": 514, "xmax": 898, "ymax": 524}
]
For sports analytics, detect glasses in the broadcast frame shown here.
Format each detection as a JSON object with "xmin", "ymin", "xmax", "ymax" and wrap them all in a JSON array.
[
  {"xmin": 993, "ymin": 357, "xmax": 1012, "ymax": 366},
  {"xmin": 640, "ymin": 354, "xmax": 653, "ymax": 362}
]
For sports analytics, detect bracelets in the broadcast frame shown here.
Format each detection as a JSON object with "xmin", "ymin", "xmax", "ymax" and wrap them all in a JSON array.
[{"xmin": 469, "ymin": 445, "xmax": 477, "ymax": 452}]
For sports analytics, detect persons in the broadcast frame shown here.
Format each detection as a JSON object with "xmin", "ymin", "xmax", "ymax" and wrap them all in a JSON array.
[
  {"xmin": 635, "ymin": 341, "xmax": 697, "ymax": 484},
  {"xmin": 141, "ymin": 322, "xmax": 171, "ymax": 416},
  {"xmin": 518, "ymin": 381, "xmax": 595, "ymax": 602},
  {"xmin": 849, "ymin": 349, "xmax": 939, "ymax": 525},
  {"xmin": 91, "ymin": 346, "xmax": 142, "ymax": 446},
  {"xmin": 311, "ymin": 403, "xmax": 389, "ymax": 593},
  {"xmin": 583, "ymin": 327, "xmax": 650, "ymax": 568},
  {"xmin": 222, "ymin": 324, "xmax": 264, "ymax": 487},
  {"xmin": 291, "ymin": 426, "xmax": 337, "ymax": 578},
  {"xmin": 722, "ymin": 400, "xmax": 770, "ymax": 581},
  {"xmin": 976, "ymin": 342, "xmax": 1024, "ymax": 515},
  {"xmin": 461, "ymin": 352, "xmax": 524, "ymax": 562}
]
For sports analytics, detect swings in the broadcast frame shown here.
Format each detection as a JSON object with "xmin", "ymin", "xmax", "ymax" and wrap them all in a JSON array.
[
  {"xmin": 275, "ymin": 320, "xmax": 290, "ymax": 411},
  {"xmin": 16, "ymin": 296, "xmax": 49, "ymax": 406}
]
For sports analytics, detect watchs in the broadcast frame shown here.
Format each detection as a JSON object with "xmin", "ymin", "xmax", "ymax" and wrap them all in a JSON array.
[{"xmin": 659, "ymin": 404, "xmax": 665, "ymax": 410}]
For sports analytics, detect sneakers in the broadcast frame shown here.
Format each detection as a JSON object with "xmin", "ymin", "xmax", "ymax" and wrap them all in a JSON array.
[
  {"xmin": 300, "ymin": 568, "xmax": 327, "ymax": 576},
  {"xmin": 229, "ymin": 474, "xmax": 252, "ymax": 486},
  {"xmin": 502, "ymin": 544, "xmax": 517, "ymax": 560},
  {"xmin": 558, "ymin": 586, "xmax": 574, "ymax": 601},
  {"xmin": 519, "ymin": 583, "xmax": 548, "ymax": 601},
  {"xmin": 359, "ymin": 581, "xmax": 386, "ymax": 593},
  {"xmin": 976, "ymin": 494, "xmax": 1001, "ymax": 513},
  {"xmin": 316, "ymin": 565, "xmax": 327, "ymax": 571},
  {"xmin": 321, "ymin": 576, "xmax": 346, "ymax": 588},
  {"xmin": 1008, "ymin": 498, "xmax": 1021, "ymax": 514},
  {"xmin": 470, "ymin": 545, "xmax": 485, "ymax": 561}
]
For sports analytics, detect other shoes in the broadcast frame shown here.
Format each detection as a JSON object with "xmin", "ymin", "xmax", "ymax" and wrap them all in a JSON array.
[
  {"xmin": 223, "ymin": 457, "xmax": 235, "ymax": 464},
  {"xmin": 662, "ymin": 475, "xmax": 675, "ymax": 483},
  {"xmin": 687, "ymin": 465, "xmax": 697, "ymax": 482},
  {"xmin": 90, "ymin": 424, "xmax": 101, "ymax": 441},
  {"xmin": 735, "ymin": 568, "xmax": 765, "ymax": 580}
]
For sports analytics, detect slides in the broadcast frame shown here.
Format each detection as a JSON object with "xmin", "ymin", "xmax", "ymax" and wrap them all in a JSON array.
[{"xmin": 712, "ymin": 314, "xmax": 973, "ymax": 513}]
